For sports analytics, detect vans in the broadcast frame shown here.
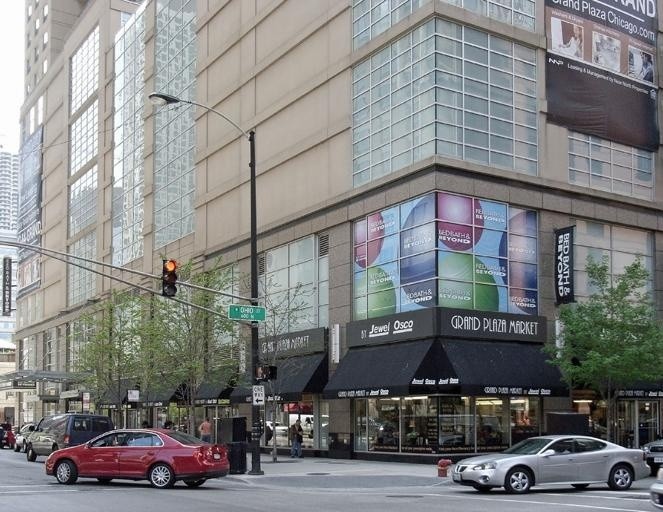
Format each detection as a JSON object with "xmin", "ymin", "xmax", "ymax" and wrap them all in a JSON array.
[{"xmin": 25, "ymin": 413, "xmax": 115, "ymax": 463}]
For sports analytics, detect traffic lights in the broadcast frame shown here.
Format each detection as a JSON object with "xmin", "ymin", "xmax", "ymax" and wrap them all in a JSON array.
[{"xmin": 161, "ymin": 258, "xmax": 177, "ymax": 297}]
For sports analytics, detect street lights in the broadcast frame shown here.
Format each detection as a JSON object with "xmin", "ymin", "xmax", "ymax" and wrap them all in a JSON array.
[{"xmin": 147, "ymin": 90, "xmax": 273, "ymax": 476}]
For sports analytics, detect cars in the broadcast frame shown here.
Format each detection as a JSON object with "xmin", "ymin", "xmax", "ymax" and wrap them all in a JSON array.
[
  {"xmin": 14, "ymin": 423, "xmax": 38, "ymax": 452},
  {"xmin": 450, "ymin": 433, "xmax": 651, "ymax": 492},
  {"xmin": 44, "ymin": 425, "xmax": 229, "ymax": 489}
]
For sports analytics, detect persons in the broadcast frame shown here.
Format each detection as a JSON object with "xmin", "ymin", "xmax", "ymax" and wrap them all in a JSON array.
[
  {"xmin": 558, "ymin": 24, "xmax": 582, "ymax": 59},
  {"xmin": 198, "ymin": 417, "xmax": 212, "ymax": 444},
  {"xmin": 0, "ymin": 426, "xmax": 5, "ymax": 449},
  {"xmin": 289, "ymin": 419, "xmax": 304, "ymax": 458},
  {"xmin": 635, "ymin": 50, "xmax": 653, "ymax": 83},
  {"xmin": 5, "ymin": 428, "xmax": 14, "ymax": 449},
  {"xmin": 522, "ymin": 415, "xmax": 530, "ymax": 425}
]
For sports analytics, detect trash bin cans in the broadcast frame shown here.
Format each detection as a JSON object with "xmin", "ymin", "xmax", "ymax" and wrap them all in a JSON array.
[{"xmin": 228, "ymin": 441, "xmax": 246, "ymax": 472}]
